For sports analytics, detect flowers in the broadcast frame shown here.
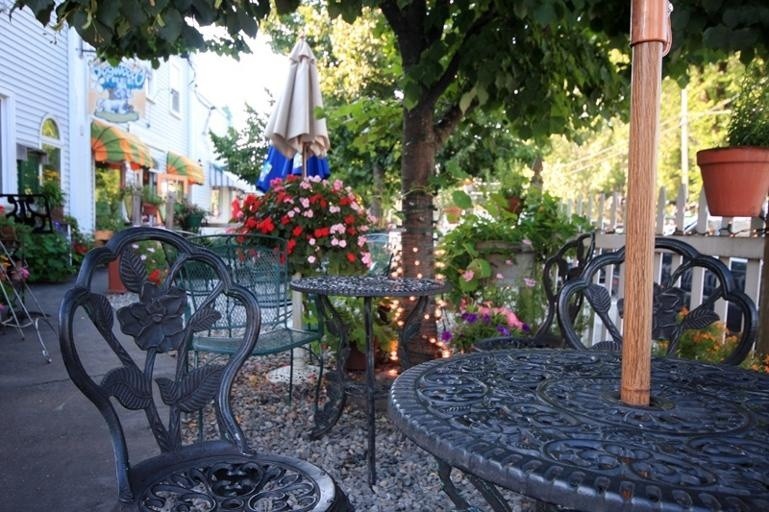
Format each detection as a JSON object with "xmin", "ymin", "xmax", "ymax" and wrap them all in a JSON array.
[{"xmin": 227, "ymin": 175, "xmax": 376, "ymax": 276}]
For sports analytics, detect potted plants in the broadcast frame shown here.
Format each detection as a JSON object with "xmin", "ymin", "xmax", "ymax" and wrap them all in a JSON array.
[
  {"xmin": 696, "ymin": 57, "xmax": 768, "ymax": 218},
  {"xmin": 437, "ymin": 182, "xmax": 594, "ymax": 333},
  {"xmin": 301, "ymin": 286, "xmax": 399, "ymax": 371},
  {"xmin": 141, "ymin": 185, "xmax": 164, "ymax": 216},
  {"xmin": 496, "ymin": 172, "xmax": 528, "ymax": 220}
]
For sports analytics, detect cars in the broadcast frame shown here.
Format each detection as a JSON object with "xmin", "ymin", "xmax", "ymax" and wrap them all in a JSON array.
[{"xmin": 598, "ymin": 214, "xmax": 765, "ymax": 332}]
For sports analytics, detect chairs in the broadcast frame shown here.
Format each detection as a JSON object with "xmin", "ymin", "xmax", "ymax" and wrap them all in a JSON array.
[
  {"xmin": 472, "ymin": 232, "xmax": 596, "ymax": 352},
  {"xmin": 157, "ymin": 234, "xmax": 325, "ymax": 445},
  {"xmin": 558, "ymin": 233, "xmax": 759, "ymax": 369},
  {"xmin": 58, "ymin": 226, "xmax": 355, "ymax": 512}
]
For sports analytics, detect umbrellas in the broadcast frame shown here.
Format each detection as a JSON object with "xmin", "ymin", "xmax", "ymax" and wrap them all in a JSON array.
[
  {"xmin": 254, "ymin": 134, "xmax": 331, "ymax": 195},
  {"xmin": 263, "ymin": 35, "xmax": 331, "ymax": 182}
]
